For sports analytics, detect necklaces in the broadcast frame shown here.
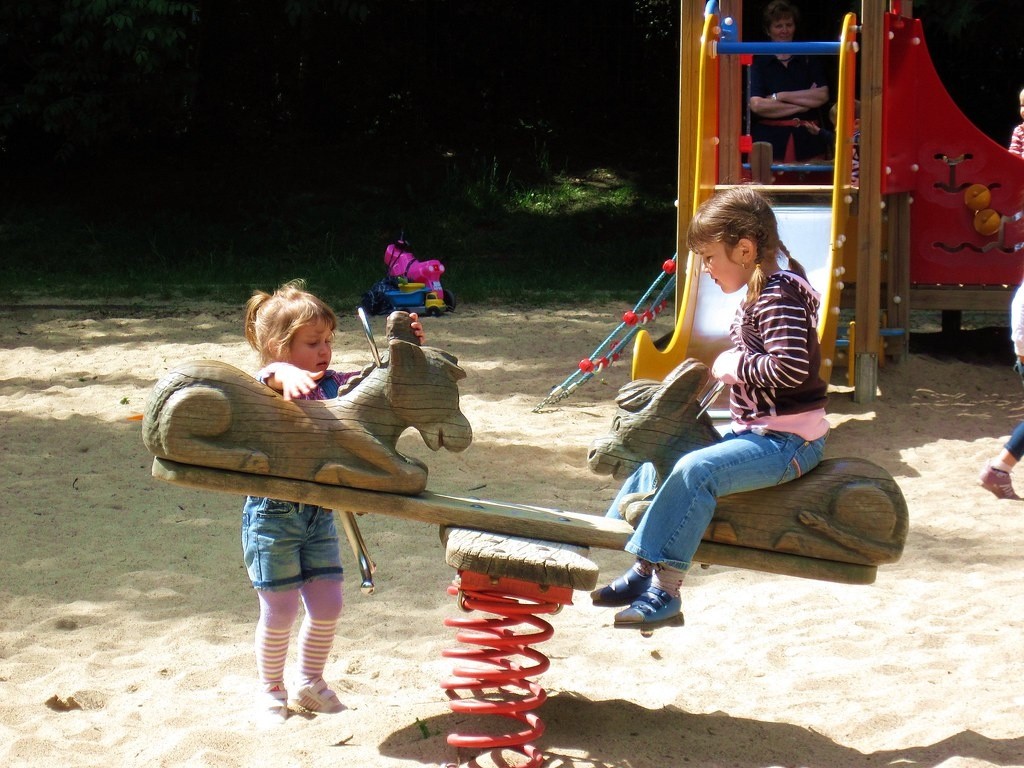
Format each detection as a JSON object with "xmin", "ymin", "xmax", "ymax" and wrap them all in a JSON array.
[{"xmin": 781, "ymin": 57, "xmax": 791, "ymax": 62}]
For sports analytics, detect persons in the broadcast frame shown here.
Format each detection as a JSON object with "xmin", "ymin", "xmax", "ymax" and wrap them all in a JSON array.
[
  {"xmin": 743, "ymin": 0, "xmax": 830, "ymax": 166},
  {"xmin": 1008, "ymin": 89, "xmax": 1024, "ymax": 158},
  {"xmin": 241, "ymin": 282, "xmax": 426, "ymax": 718},
  {"xmin": 830, "ymin": 98, "xmax": 859, "ymax": 185},
  {"xmin": 588, "ymin": 190, "xmax": 830, "ymax": 628},
  {"xmin": 976, "ymin": 282, "xmax": 1024, "ymax": 501}
]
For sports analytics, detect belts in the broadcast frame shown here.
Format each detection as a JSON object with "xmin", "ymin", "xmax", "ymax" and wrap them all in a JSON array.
[{"xmin": 760, "ymin": 118, "xmax": 819, "ymax": 128}]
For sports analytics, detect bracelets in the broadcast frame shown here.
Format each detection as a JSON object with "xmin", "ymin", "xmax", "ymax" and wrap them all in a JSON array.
[{"xmin": 772, "ymin": 93, "xmax": 777, "ymax": 100}]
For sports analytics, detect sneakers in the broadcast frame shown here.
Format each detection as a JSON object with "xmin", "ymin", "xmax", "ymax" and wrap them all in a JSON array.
[
  {"xmin": 590, "ymin": 566, "xmax": 651, "ymax": 602},
  {"xmin": 614, "ymin": 587, "xmax": 682, "ymax": 623},
  {"xmin": 978, "ymin": 466, "xmax": 1020, "ymax": 500},
  {"xmin": 293, "ymin": 680, "xmax": 346, "ymax": 712},
  {"xmin": 263, "ymin": 689, "xmax": 288, "ymax": 724}
]
